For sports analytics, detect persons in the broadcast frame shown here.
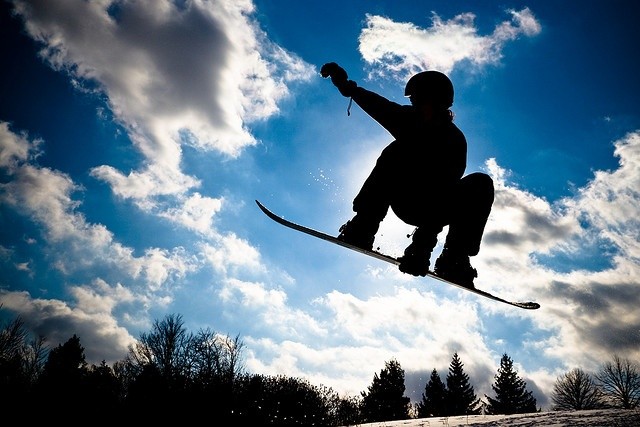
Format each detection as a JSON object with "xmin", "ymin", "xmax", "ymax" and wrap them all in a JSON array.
[{"xmin": 319, "ymin": 61, "xmax": 494, "ymax": 288}]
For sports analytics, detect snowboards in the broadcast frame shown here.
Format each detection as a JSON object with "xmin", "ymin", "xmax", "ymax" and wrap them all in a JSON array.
[{"xmin": 255, "ymin": 199, "xmax": 540, "ymax": 310}]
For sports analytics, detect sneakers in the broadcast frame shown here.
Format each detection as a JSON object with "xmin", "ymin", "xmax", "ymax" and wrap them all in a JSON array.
[
  {"xmin": 434, "ymin": 249, "xmax": 477, "ymax": 289},
  {"xmin": 337, "ymin": 215, "xmax": 379, "ymax": 251}
]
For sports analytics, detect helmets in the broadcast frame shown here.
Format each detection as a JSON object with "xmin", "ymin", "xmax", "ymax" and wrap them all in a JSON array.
[{"xmin": 404, "ymin": 71, "xmax": 454, "ymax": 107}]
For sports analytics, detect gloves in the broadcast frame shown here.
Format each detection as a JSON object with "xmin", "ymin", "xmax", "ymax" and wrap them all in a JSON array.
[
  {"xmin": 320, "ymin": 62, "xmax": 357, "ymax": 97},
  {"xmin": 398, "ymin": 228, "xmax": 437, "ymax": 277}
]
